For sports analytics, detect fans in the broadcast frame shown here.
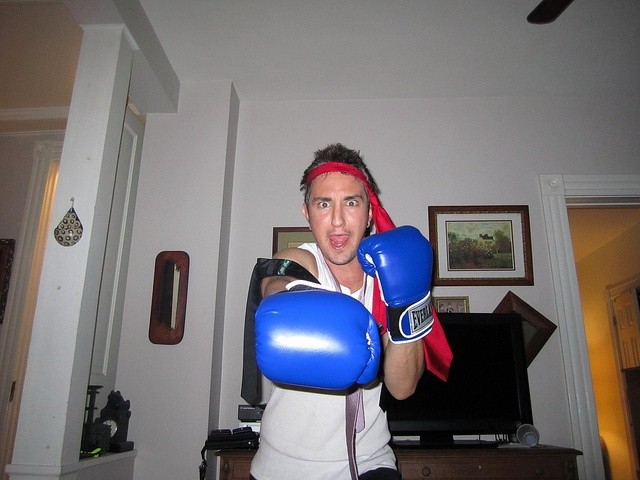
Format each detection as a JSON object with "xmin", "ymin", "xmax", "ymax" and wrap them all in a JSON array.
[{"xmin": 527, "ymin": 0, "xmax": 573, "ymax": 24}]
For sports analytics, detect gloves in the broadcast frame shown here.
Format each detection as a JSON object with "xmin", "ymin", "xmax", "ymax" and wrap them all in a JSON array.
[
  {"xmin": 358, "ymin": 224, "xmax": 435, "ymax": 346},
  {"xmin": 253, "ymin": 285, "xmax": 382, "ymax": 391}
]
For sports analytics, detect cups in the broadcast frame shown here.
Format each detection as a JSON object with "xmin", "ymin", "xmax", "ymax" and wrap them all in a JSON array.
[{"xmin": 517, "ymin": 424, "xmax": 540, "ymax": 447}]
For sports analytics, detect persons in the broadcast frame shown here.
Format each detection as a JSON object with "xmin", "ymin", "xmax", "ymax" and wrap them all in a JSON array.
[{"xmin": 249, "ymin": 142, "xmax": 435, "ymax": 479}]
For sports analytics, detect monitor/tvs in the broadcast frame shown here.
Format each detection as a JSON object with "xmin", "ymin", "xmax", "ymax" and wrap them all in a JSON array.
[{"xmin": 380, "ymin": 313, "xmax": 534, "ymax": 448}]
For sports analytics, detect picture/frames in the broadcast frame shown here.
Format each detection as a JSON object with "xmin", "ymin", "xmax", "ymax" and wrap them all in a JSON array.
[
  {"xmin": 431, "ymin": 296, "xmax": 469, "ymax": 313},
  {"xmin": 428, "ymin": 205, "xmax": 534, "ymax": 286},
  {"xmin": 492, "ymin": 291, "xmax": 558, "ymax": 370},
  {"xmin": 273, "ymin": 227, "xmax": 318, "ymax": 257}
]
